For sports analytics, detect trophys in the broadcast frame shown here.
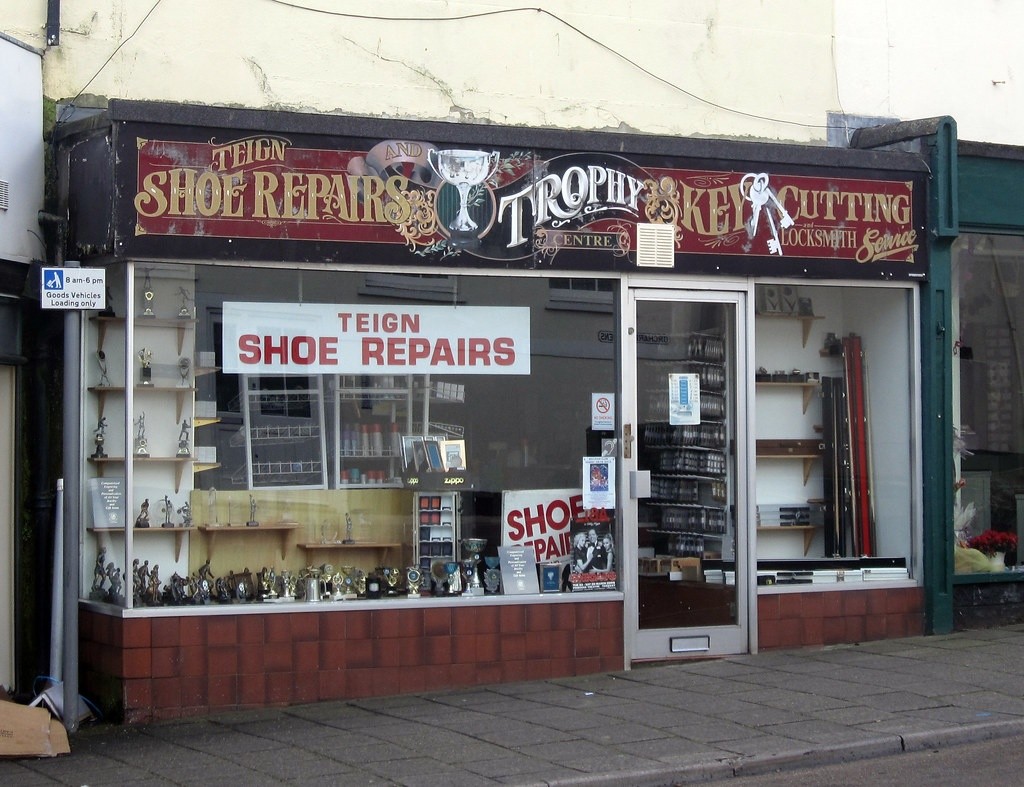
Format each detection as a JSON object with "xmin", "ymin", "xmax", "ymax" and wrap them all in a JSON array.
[
  {"xmin": 259, "ymin": 537, "xmax": 501, "ymax": 597},
  {"xmin": 427, "ymin": 147, "xmax": 501, "ymax": 232}
]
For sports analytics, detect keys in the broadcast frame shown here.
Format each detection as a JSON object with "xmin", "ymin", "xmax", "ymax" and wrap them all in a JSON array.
[{"xmin": 747, "ymin": 182, "xmax": 795, "ymax": 257}]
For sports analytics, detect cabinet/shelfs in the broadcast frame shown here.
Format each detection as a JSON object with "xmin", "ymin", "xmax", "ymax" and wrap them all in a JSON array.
[
  {"xmin": 412, "ymin": 491, "xmax": 461, "ymax": 572},
  {"xmin": 194, "ymin": 367, "xmax": 221, "ymax": 472},
  {"xmin": 643, "ymin": 304, "xmax": 736, "ymax": 562},
  {"xmin": 239, "ymin": 374, "xmax": 328, "ymax": 491},
  {"xmin": 640, "ymin": 576, "xmax": 735, "ymax": 629},
  {"xmin": 88, "ymin": 315, "xmax": 198, "ymax": 563},
  {"xmin": 756, "ymin": 312, "xmax": 826, "ymax": 556},
  {"xmin": 328, "ymin": 373, "xmax": 466, "ymax": 491}
]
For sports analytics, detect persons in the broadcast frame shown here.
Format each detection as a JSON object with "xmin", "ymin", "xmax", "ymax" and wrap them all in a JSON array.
[
  {"xmin": 346, "ymin": 513, "xmax": 353, "ymax": 539},
  {"xmin": 139, "ymin": 266, "xmax": 156, "ymax": 289},
  {"xmin": 571, "ymin": 528, "xmax": 615, "ymax": 573},
  {"xmin": 249, "ymin": 494, "xmax": 256, "ymax": 522},
  {"xmin": 86, "ymin": 410, "xmax": 213, "ymax": 604},
  {"xmin": 174, "ymin": 286, "xmax": 194, "ymax": 304}
]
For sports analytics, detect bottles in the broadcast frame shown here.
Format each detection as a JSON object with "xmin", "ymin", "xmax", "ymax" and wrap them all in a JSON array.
[
  {"xmin": 343, "ymin": 423, "xmax": 358, "ymax": 455},
  {"xmin": 389, "ymin": 424, "xmax": 401, "ymax": 457},
  {"xmin": 360, "ymin": 424, "xmax": 369, "ymax": 455},
  {"xmin": 372, "ymin": 425, "xmax": 382, "ymax": 455},
  {"xmin": 340, "ymin": 464, "xmax": 387, "ymax": 484}
]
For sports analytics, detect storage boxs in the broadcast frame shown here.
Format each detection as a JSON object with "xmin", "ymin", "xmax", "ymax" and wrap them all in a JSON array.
[
  {"xmin": 413, "ymin": 381, "xmax": 464, "ymax": 401},
  {"xmin": 194, "ymin": 447, "xmax": 216, "ymax": 463},
  {"xmin": 195, "ymin": 401, "xmax": 216, "ymax": 418},
  {"xmin": 402, "ymin": 470, "xmax": 470, "ymax": 490},
  {"xmin": 195, "ymin": 352, "xmax": 215, "ymax": 368},
  {"xmin": 703, "ymin": 551, "xmax": 721, "ymax": 559}
]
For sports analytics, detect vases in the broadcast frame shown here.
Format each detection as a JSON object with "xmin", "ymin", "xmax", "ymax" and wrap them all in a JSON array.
[{"xmin": 988, "ymin": 552, "xmax": 1007, "ymax": 565}]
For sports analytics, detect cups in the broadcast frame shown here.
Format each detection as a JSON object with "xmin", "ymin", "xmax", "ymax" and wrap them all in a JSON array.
[
  {"xmin": 366, "ymin": 577, "xmax": 381, "ymax": 599},
  {"xmin": 306, "ymin": 578, "xmax": 327, "ymax": 602}
]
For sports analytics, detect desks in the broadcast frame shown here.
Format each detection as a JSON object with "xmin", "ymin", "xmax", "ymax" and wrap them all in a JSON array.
[{"xmin": 961, "ymin": 469, "xmax": 992, "ymax": 532}]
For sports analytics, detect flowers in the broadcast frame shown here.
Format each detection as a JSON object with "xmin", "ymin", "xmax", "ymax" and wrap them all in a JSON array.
[{"xmin": 968, "ymin": 530, "xmax": 1019, "ymax": 557}]
